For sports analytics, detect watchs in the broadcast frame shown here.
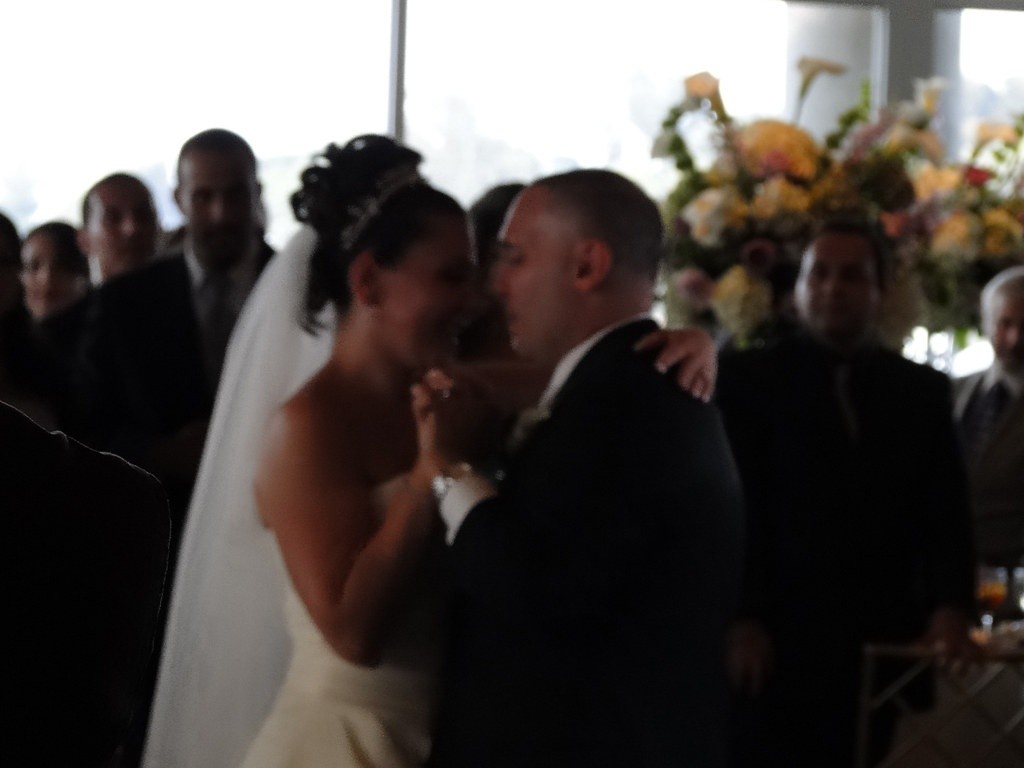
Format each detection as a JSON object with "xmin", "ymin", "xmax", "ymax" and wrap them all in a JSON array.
[{"xmin": 432, "ymin": 457, "xmax": 488, "ymax": 497}]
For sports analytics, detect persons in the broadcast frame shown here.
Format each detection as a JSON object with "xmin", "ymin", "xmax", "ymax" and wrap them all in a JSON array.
[{"xmin": 1, "ymin": 129, "xmax": 1023, "ymax": 768}]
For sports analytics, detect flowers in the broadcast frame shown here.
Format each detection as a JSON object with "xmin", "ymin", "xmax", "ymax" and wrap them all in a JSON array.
[
  {"xmin": 906, "ymin": 75, "xmax": 1024, "ymax": 350},
  {"xmin": 650, "ymin": 51, "xmax": 946, "ymax": 345}
]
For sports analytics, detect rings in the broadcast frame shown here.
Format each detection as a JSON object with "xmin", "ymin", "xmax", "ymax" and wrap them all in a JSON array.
[{"xmin": 433, "ymin": 389, "xmax": 465, "ymax": 407}]
